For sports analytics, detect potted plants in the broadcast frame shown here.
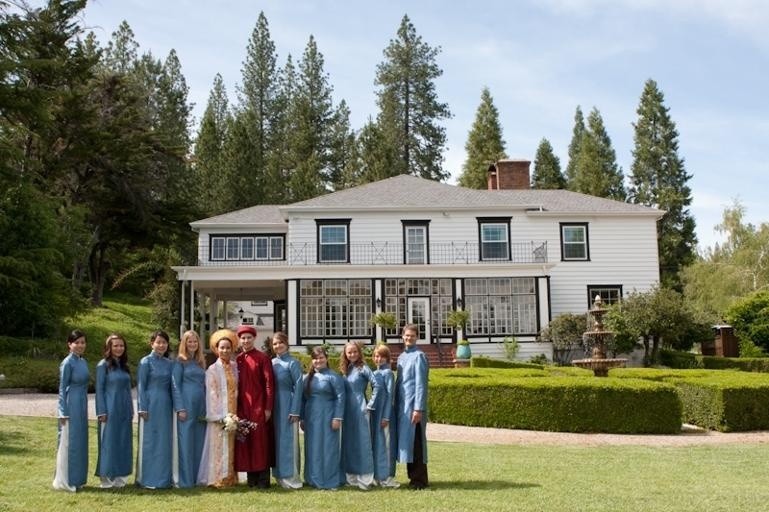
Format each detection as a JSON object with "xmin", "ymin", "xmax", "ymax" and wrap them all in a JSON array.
[{"xmin": 455, "ymin": 340, "xmax": 471, "ymax": 360}]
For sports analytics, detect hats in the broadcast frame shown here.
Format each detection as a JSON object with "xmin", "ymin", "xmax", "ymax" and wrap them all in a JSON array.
[
  {"xmin": 237, "ymin": 325, "xmax": 257, "ymax": 337},
  {"xmin": 210, "ymin": 329, "xmax": 238, "ymax": 356}
]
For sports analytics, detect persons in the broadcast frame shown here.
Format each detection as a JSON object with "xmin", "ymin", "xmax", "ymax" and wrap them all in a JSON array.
[
  {"xmin": 395, "ymin": 324, "xmax": 429, "ymax": 489},
  {"xmin": 339, "ymin": 341, "xmax": 379, "ymax": 491},
  {"xmin": 52, "ymin": 331, "xmax": 91, "ymax": 491},
  {"xmin": 204, "ymin": 329, "xmax": 240, "ymax": 491},
  {"xmin": 371, "ymin": 345, "xmax": 398, "ymax": 486},
  {"xmin": 236, "ymin": 326, "xmax": 275, "ymax": 489},
  {"xmin": 271, "ymin": 331, "xmax": 303, "ymax": 490},
  {"xmin": 135, "ymin": 330, "xmax": 173, "ymax": 490},
  {"xmin": 300, "ymin": 345, "xmax": 346, "ymax": 489},
  {"xmin": 171, "ymin": 329, "xmax": 206, "ymax": 489},
  {"xmin": 94, "ymin": 334, "xmax": 134, "ymax": 488}
]
